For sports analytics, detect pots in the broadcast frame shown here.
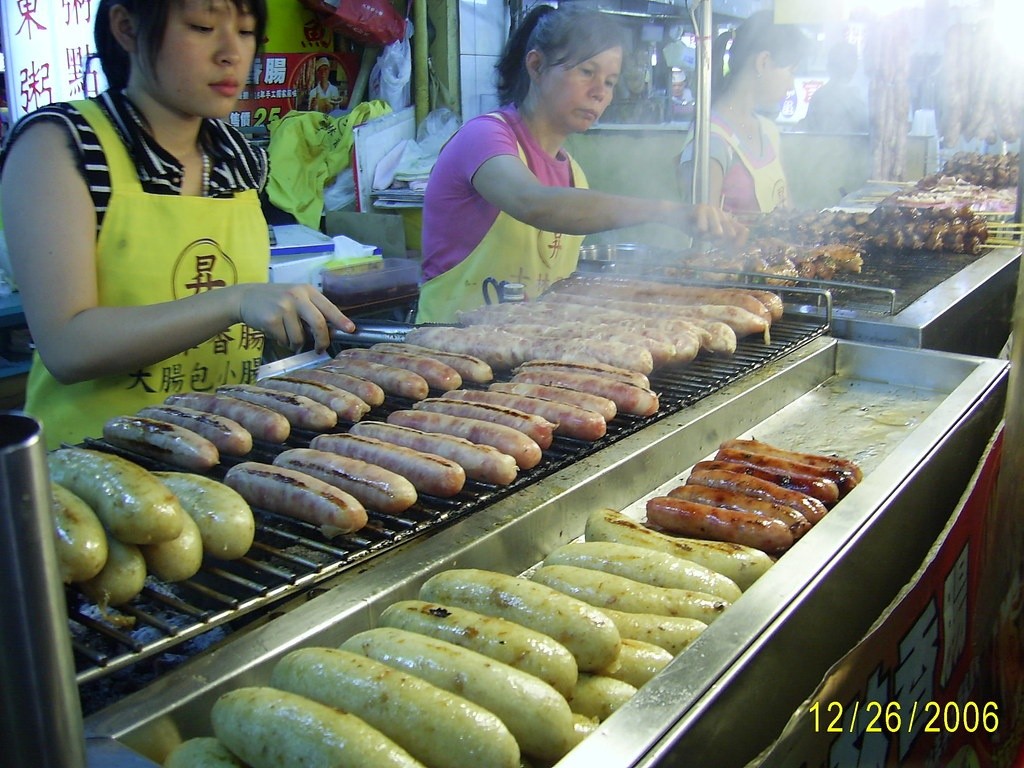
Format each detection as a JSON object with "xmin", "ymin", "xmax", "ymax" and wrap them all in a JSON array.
[{"xmin": 577, "ymin": 242, "xmax": 675, "ymax": 273}]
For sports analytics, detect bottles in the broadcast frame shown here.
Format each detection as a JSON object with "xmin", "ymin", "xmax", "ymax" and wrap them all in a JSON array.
[{"xmin": 502, "ymin": 283, "xmax": 525, "ymax": 303}]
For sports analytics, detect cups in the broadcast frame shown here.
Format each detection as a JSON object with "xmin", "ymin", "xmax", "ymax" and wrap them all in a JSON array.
[
  {"xmin": 0, "ymin": 412, "xmax": 90, "ymax": 768},
  {"xmin": 907, "ymin": 109, "xmax": 937, "ymax": 137}
]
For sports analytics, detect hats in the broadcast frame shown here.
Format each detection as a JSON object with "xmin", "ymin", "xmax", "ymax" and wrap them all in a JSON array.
[{"xmin": 316, "ymin": 57, "xmax": 330, "ymax": 71}]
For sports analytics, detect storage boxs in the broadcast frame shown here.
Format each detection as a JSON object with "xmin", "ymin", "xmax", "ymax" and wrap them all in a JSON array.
[{"xmin": 319, "ymin": 257, "xmax": 422, "ymax": 294}]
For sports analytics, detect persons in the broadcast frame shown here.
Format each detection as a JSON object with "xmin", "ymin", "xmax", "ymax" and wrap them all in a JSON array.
[
  {"xmin": 308, "ymin": 57, "xmax": 340, "ymax": 113},
  {"xmin": 0, "ymin": 0, "xmax": 356, "ymax": 453},
  {"xmin": 679, "ymin": 13, "xmax": 816, "ymax": 254},
  {"xmin": 414, "ymin": 5, "xmax": 749, "ymax": 328},
  {"xmin": 653, "ymin": 70, "xmax": 693, "ymax": 116},
  {"xmin": 804, "ymin": 44, "xmax": 872, "ymax": 136}
]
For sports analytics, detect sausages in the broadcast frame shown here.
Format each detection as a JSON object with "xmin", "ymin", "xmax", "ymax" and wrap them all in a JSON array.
[
  {"xmin": 38, "ymin": 277, "xmax": 783, "ymax": 606},
  {"xmin": 165, "ymin": 434, "xmax": 862, "ymax": 768}
]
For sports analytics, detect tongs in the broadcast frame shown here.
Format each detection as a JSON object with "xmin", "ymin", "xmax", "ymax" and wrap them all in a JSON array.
[{"xmin": 301, "ymin": 318, "xmax": 468, "ymax": 347}]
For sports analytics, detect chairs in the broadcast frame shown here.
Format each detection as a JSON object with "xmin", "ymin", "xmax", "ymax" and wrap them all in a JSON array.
[{"xmin": 324, "ymin": 211, "xmax": 408, "ymax": 258}]
